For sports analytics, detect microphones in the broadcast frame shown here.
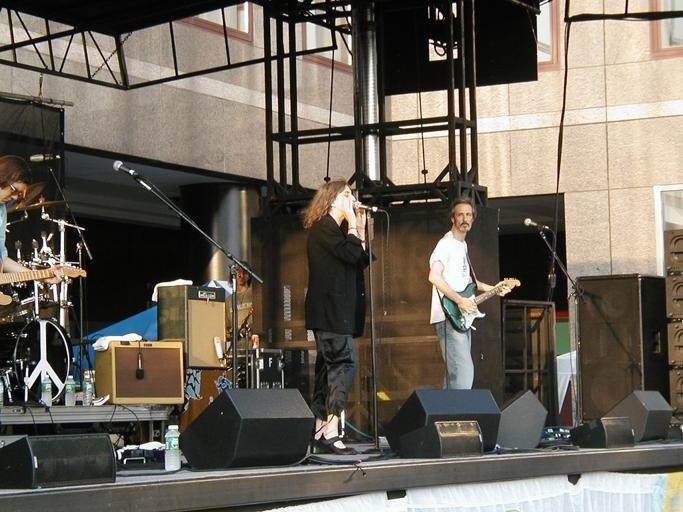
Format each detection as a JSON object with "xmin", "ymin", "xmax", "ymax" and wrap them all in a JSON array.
[
  {"xmin": 135, "ymin": 353, "xmax": 148, "ymax": 379},
  {"xmin": 353, "ymin": 198, "xmax": 377, "ymax": 214},
  {"xmin": 523, "ymin": 216, "xmax": 550, "ymax": 232},
  {"xmin": 110, "ymin": 158, "xmax": 139, "ymax": 180},
  {"xmin": 27, "ymin": 151, "xmax": 63, "ymax": 164},
  {"xmin": 213, "ymin": 336, "xmax": 224, "ymax": 363}
]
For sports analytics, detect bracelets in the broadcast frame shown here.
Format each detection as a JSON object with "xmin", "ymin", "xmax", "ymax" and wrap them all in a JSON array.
[
  {"xmin": 360, "ymin": 239, "xmax": 367, "ymax": 243},
  {"xmin": 348, "ymin": 226, "xmax": 357, "ymax": 231}
]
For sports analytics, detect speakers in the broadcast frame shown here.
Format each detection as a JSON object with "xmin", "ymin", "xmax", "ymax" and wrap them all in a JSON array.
[
  {"xmin": 598, "ymin": 389, "xmax": 673, "ymax": 442},
  {"xmin": 157, "ymin": 286, "xmax": 228, "ymax": 370},
  {"xmin": 383, "ymin": 388, "xmax": 501, "ymax": 458},
  {"xmin": 576, "ymin": 273, "xmax": 671, "ymax": 422},
  {"xmin": 497, "ymin": 387, "xmax": 549, "ymax": 449},
  {"xmin": 178, "ymin": 387, "xmax": 316, "ymax": 467}
]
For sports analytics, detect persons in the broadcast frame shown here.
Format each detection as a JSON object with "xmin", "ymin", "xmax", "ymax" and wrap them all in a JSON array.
[
  {"xmin": 224, "ymin": 259, "xmax": 255, "ymax": 342},
  {"xmin": 0, "ymin": 154, "xmax": 66, "ymax": 303},
  {"xmin": 299, "ymin": 179, "xmax": 378, "ymax": 457},
  {"xmin": 425, "ymin": 198, "xmax": 512, "ymax": 390}
]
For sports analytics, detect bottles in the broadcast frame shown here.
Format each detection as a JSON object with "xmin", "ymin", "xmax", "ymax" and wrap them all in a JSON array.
[
  {"xmin": 64, "ymin": 375, "xmax": 75, "ymax": 406},
  {"xmin": 82, "ymin": 375, "xmax": 92, "ymax": 405},
  {"xmin": 174, "ymin": 425, "xmax": 180, "ymax": 469},
  {"xmin": 164, "ymin": 424, "xmax": 179, "ymax": 470},
  {"xmin": 40, "ymin": 375, "xmax": 51, "ymax": 407}
]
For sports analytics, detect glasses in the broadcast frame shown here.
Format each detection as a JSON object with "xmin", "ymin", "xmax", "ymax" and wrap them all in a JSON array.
[{"xmin": 4, "ymin": 178, "xmax": 24, "ymax": 199}]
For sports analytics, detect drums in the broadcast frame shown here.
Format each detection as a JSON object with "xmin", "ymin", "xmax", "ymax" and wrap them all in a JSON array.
[
  {"xmin": 0, "ymin": 310, "xmax": 75, "ymax": 406},
  {"xmin": 10, "ymin": 259, "xmax": 60, "ymax": 304}
]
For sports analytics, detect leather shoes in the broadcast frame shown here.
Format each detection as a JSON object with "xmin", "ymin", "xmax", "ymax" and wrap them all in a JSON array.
[{"xmin": 316, "ymin": 432, "xmax": 356, "ymax": 454}]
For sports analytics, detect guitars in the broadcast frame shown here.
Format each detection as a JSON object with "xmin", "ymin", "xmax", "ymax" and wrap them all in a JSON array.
[
  {"xmin": 0, "ymin": 257, "xmax": 87, "ymax": 306},
  {"xmin": 442, "ymin": 278, "xmax": 521, "ymax": 332}
]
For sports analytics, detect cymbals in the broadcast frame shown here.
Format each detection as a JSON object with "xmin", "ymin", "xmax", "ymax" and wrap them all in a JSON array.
[
  {"xmin": 16, "ymin": 201, "xmax": 67, "ymax": 211},
  {"xmin": 6, "ymin": 181, "xmax": 47, "ymax": 213}
]
